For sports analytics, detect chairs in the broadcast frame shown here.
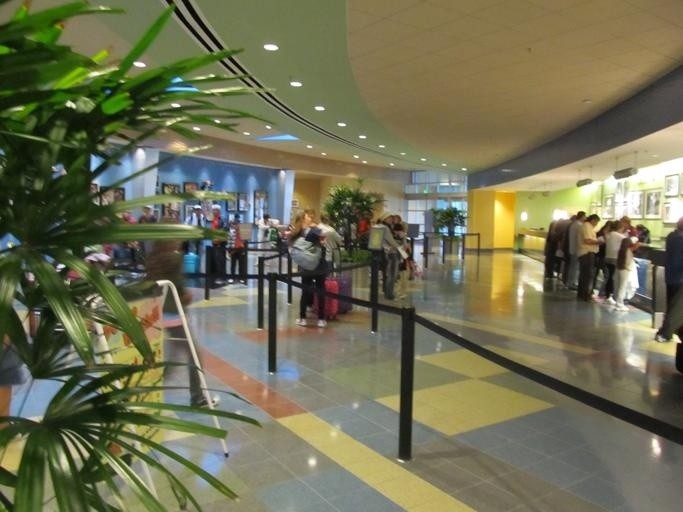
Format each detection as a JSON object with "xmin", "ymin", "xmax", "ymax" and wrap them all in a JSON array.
[{"xmin": 405, "ymin": 224, "xmax": 419, "ymax": 247}]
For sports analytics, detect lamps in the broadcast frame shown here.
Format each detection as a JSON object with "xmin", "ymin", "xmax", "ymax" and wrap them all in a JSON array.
[{"xmin": 577, "ymin": 151, "xmax": 639, "ymax": 188}]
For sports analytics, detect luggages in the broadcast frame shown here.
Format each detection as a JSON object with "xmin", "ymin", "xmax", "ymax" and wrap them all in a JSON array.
[
  {"xmin": 312, "ymin": 276, "xmax": 353, "ymax": 317},
  {"xmin": 183, "ymin": 252, "xmax": 200, "ymax": 274}
]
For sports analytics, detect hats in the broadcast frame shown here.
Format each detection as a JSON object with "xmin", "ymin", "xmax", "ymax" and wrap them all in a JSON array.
[{"xmin": 379, "ymin": 211, "xmax": 393, "ymax": 221}]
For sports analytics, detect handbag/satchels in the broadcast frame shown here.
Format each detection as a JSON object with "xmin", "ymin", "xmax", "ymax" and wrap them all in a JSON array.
[{"xmin": 288, "ymin": 236, "xmax": 324, "ymax": 271}]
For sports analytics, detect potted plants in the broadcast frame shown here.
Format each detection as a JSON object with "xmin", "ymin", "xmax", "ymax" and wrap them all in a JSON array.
[
  {"xmin": 321, "ymin": 177, "xmax": 389, "ymax": 287},
  {"xmin": 430, "ymin": 208, "xmax": 471, "ymax": 255}
]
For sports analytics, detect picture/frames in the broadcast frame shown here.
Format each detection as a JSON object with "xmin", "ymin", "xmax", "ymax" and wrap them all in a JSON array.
[
  {"xmin": 589, "ymin": 172, "xmax": 683, "ymax": 224},
  {"xmin": 100, "ymin": 186, "xmax": 125, "ymax": 207},
  {"xmin": 154, "ymin": 174, "xmax": 269, "ymax": 225}
]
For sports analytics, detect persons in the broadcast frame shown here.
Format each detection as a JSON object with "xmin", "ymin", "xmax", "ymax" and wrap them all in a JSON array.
[
  {"xmin": 116, "ymin": 202, "xmax": 246, "ymax": 285},
  {"xmin": 541, "ymin": 211, "xmax": 683, "ymax": 377},
  {"xmin": 370, "ymin": 209, "xmax": 413, "ymax": 298},
  {"xmin": 258, "ymin": 209, "xmax": 343, "ymax": 328}
]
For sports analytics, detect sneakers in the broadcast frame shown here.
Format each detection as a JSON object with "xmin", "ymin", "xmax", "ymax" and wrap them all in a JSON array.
[
  {"xmin": 316, "ymin": 319, "xmax": 328, "ymax": 327},
  {"xmin": 295, "ymin": 318, "xmax": 308, "ymax": 327},
  {"xmin": 567, "ymin": 282, "xmax": 630, "ymax": 312}
]
